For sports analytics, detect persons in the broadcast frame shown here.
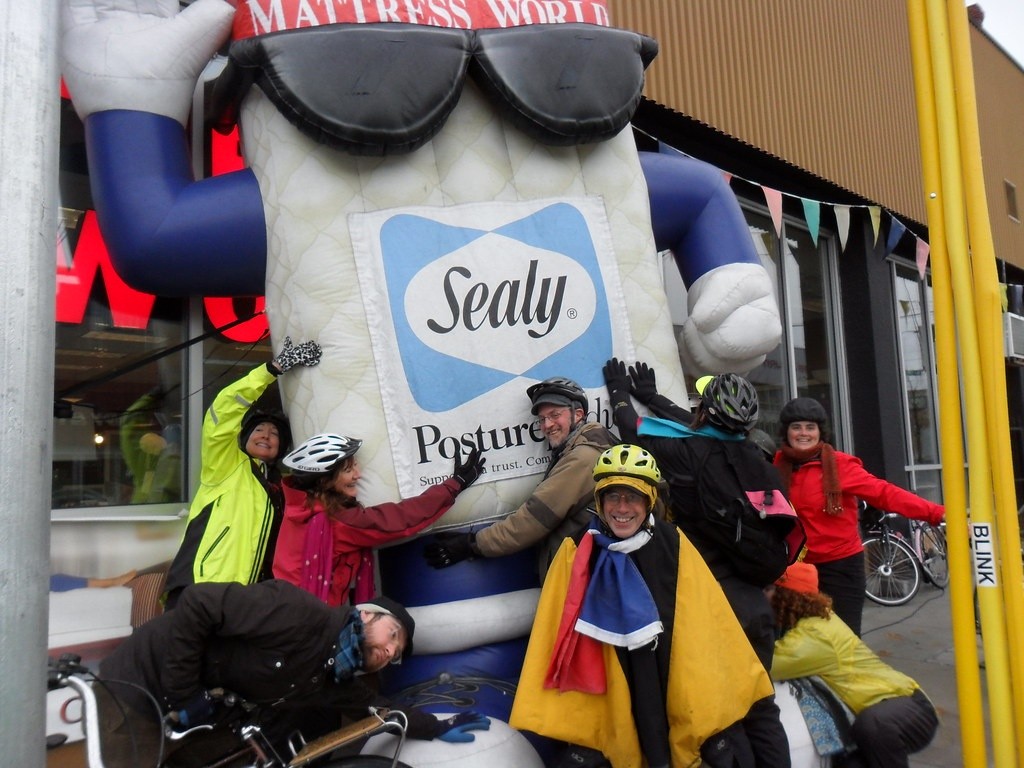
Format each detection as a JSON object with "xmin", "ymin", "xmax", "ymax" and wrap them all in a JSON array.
[
  {"xmin": 509, "ymin": 445, "xmax": 774, "ymax": 768},
  {"xmin": 83, "ymin": 580, "xmax": 438, "ymax": 768},
  {"xmin": 777, "ymin": 399, "xmax": 972, "ymax": 638},
  {"xmin": 121, "ymin": 377, "xmax": 182, "ymax": 506},
  {"xmin": 427, "ymin": 377, "xmax": 617, "ymax": 768},
  {"xmin": 272, "ymin": 435, "xmax": 486, "ymax": 605},
  {"xmin": 603, "ymin": 357, "xmax": 792, "ymax": 768},
  {"xmin": 770, "ymin": 561, "xmax": 939, "ymax": 768},
  {"xmin": 158, "ymin": 337, "xmax": 324, "ymax": 613}
]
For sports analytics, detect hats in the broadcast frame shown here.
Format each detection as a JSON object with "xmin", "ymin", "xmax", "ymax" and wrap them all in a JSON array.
[
  {"xmin": 696, "ymin": 374, "xmax": 748, "ymax": 422},
  {"xmin": 236, "ymin": 408, "xmax": 291, "ymax": 460},
  {"xmin": 779, "ymin": 397, "xmax": 829, "ymax": 432},
  {"xmin": 530, "ymin": 394, "xmax": 582, "ymax": 416},
  {"xmin": 774, "ymin": 561, "xmax": 820, "ymax": 597},
  {"xmin": 747, "ymin": 427, "xmax": 777, "ymax": 457},
  {"xmin": 594, "ymin": 476, "xmax": 657, "ymax": 525},
  {"xmin": 353, "ymin": 597, "xmax": 415, "ymax": 672}
]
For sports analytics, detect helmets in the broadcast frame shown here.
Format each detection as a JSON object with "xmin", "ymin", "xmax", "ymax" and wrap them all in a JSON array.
[
  {"xmin": 282, "ymin": 433, "xmax": 363, "ymax": 477},
  {"xmin": 526, "ymin": 376, "xmax": 588, "ymax": 416},
  {"xmin": 695, "ymin": 372, "xmax": 760, "ymax": 435},
  {"xmin": 591, "ymin": 444, "xmax": 662, "ymax": 524}
]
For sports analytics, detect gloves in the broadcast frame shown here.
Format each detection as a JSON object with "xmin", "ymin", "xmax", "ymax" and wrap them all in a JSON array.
[
  {"xmin": 433, "ymin": 708, "xmax": 490, "ymax": 743},
  {"xmin": 169, "ymin": 687, "xmax": 218, "ymax": 733},
  {"xmin": 627, "ymin": 361, "xmax": 657, "ymax": 407},
  {"xmin": 273, "ymin": 336, "xmax": 323, "ymax": 375},
  {"xmin": 602, "ymin": 358, "xmax": 632, "ymax": 412},
  {"xmin": 422, "ymin": 531, "xmax": 481, "ymax": 570},
  {"xmin": 452, "ymin": 447, "xmax": 486, "ymax": 491}
]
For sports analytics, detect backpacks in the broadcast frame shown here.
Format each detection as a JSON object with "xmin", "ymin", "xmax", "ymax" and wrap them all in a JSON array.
[{"xmin": 691, "ymin": 432, "xmax": 799, "ymax": 573}]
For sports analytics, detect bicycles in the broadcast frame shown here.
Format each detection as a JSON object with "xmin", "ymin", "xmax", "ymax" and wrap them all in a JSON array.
[
  {"xmin": 858, "ymin": 500, "xmax": 950, "ymax": 606},
  {"xmin": 45, "ymin": 653, "xmax": 414, "ymax": 768}
]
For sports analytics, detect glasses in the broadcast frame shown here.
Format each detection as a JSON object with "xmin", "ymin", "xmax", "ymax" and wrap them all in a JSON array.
[
  {"xmin": 387, "ymin": 626, "xmax": 402, "ymax": 666},
  {"xmin": 601, "ymin": 492, "xmax": 647, "ymax": 506},
  {"xmin": 535, "ymin": 406, "xmax": 572, "ymax": 426}
]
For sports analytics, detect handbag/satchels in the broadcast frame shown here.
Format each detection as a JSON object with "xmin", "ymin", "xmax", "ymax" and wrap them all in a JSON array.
[{"xmin": 786, "ymin": 674, "xmax": 859, "ymax": 759}]
